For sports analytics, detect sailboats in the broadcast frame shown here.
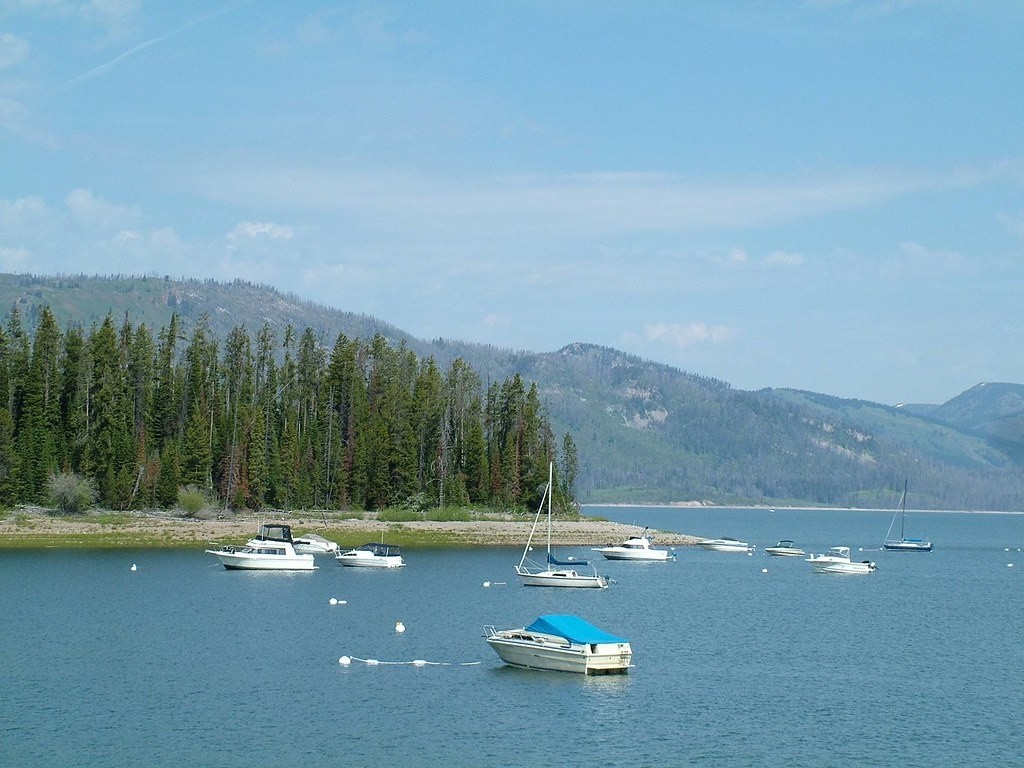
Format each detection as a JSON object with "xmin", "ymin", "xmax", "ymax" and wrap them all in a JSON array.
[
  {"xmin": 515, "ymin": 462, "xmax": 620, "ymax": 589},
  {"xmin": 882, "ymin": 479, "xmax": 934, "ymax": 552}
]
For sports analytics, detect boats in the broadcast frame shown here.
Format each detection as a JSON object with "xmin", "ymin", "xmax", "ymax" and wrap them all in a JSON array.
[
  {"xmin": 804, "ymin": 545, "xmax": 879, "ymax": 574},
  {"xmin": 334, "ymin": 542, "xmax": 407, "ymax": 569},
  {"xmin": 764, "ymin": 540, "xmax": 806, "ymax": 557},
  {"xmin": 292, "ymin": 533, "xmax": 341, "ymax": 554},
  {"xmin": 482, "ymin": 613, "xmax": 635, "ymax": 675},
  {"xmin": 204, "ymin": 523, "xmax": 321, "ymax": 571},
  {"xmin": 590, "ymin": 527, "xmax": 676, "ymax": 563},
  {"xmin": 697, "ymin": 536, "xmax": 752, "ymax": 552}
]
